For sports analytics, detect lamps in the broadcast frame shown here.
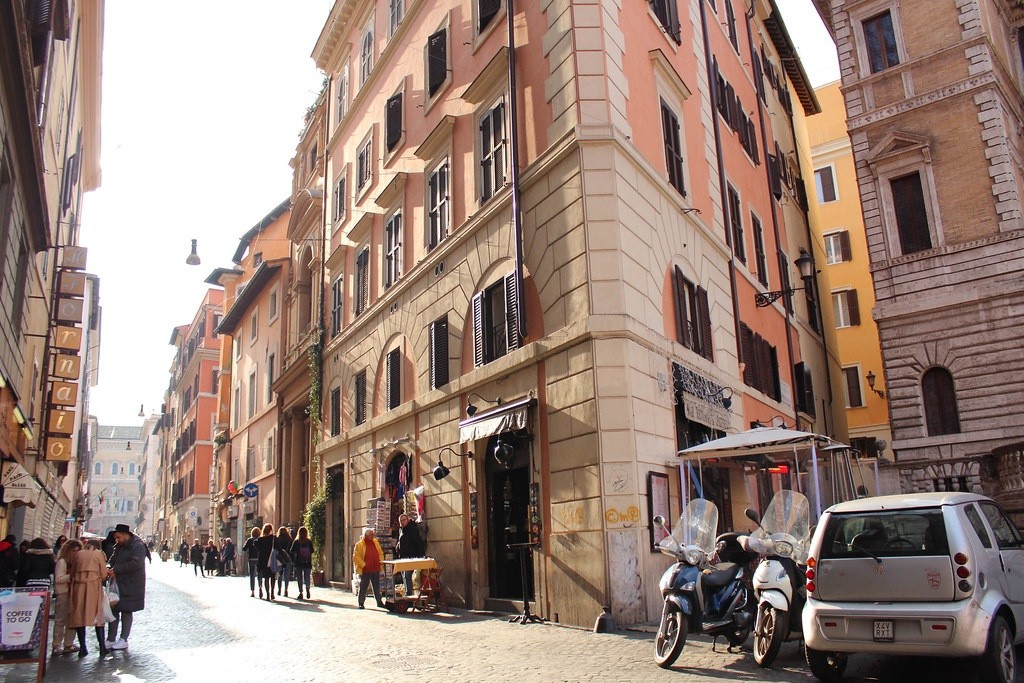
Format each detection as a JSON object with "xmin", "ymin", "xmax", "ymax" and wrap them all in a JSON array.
[
  {"xmin": 14, "ymin": 405, "xmax": 34, "ymax": 440},
  {"xmin": 139, "ymin": 404, "xmax": 145, "ymax": 417},
  {"xmin": 709, "ymin": 386, "xmax": 733, "ymax": 410},
  {"xmin": 186, "ymin": 239, "xmax": 201, "ymax": 265},
  {"xmin": 866, "ymin": 370, "xmax": 885, "ymax": 399},
  {"xmin": 754, "ymin": 246, "xmax": 815, "ymax": 307},
  {"xmin": 751, "ymin": 416, "xmax": 806, "ymax": 433},
  {"xmin": 120, "ymin": 468, "xmax": 124, "ymax": 474},
  {"xmin": 125, "ymin": 441, "xmax": 132, "ymax": 451}
]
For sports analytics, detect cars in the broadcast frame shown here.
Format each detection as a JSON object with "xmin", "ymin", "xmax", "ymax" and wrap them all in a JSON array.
[{"xmin": 801, "ymin": 490, "xmax": 1024, "ymax": 683}]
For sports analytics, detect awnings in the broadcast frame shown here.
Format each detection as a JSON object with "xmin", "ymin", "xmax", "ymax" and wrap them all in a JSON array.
[
  {"xmin": 0, "ymin": 460, "xmax": 38, "ymax": 509},
  {"xmin": 459, "ymin": 399, "xmax": 537, "ymax": 443}
]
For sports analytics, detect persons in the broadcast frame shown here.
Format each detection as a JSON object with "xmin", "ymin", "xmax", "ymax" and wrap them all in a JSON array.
[
  {"xmin": 2, "ymin": 534, "xmax": 67, "ymax": 598},
  {"xmin": 290, "ymin": 527, "xmax": 314, "ymax": 599},
  {"xmin": 396, "ymin": 514, "xmax": 424, "ymax": 596},
  {"xmin": 190, "ymin": 539, "xmax": 205, "ymax": 578},
  {"xmin": 51, "ymin": 524, "xmax": 151, "ymax": 660},
  {"xmin": 179, "ymin": 539, "xmax": 189, "ymax": 567},
  {"xmin": 205, "ymin": 538, "xmax": 235, "ymax": 576},
  {"xmin": 159, "ymin": 540, "xmax": 169, "ymax": 554},
  {"xmin": 144, "ymin": 539, "xmax": 155, "ymax": 551},
  {"xmin": 354, "ymin": 529, "xmax": 386, "ymax": 608},
  {"xmin": 243, "ymin": 524, "xmax": 293, "ymax": 600}
]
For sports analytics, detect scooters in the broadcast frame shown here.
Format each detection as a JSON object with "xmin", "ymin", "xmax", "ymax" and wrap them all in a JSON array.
[
  {"xmin": 735, "ymin": 489, "xmax": 818, "ymax": 669},
  {"xmin": 653, "ymin": 497, "xmax": 756, "ymax": 668}
]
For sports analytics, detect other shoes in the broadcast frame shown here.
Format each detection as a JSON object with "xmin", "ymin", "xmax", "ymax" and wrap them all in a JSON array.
[
  {"xmin": 267, "ymin": 596, "xmax": 269, "ymax": 600},
  {"xmin": 63, "ymin": 645, "xmax": 79, "ymax": 652},
  {"xmin": 271, "ymin": 596, "xmax": 275, "ymax": 599},
  {"xmin": 278, "ymin": 591, "xmax": 281, "ymax": 595},
  {"xmin": 377, "ymin": 602, "xmax": 384, "ymax": 607},
  {"xmin": 251, "ymin": 594, "xmax": 254, "ymax": 597},
  {"xmin": 79, "ymin": 649, "xmax": 88, "ymax": 657},
  {"xmin": 359, "ymin": 604, "xmax": 364, "ymax": 609},
  {"xmin": 100, "ymin": 648, "xmax": 110, "ymax": 655},
  {"xmin": 285, "ymin": 591, "xmax": 288, "ymax": 597},
  {"xmin": 307, "ymin": 593, "xmax": 310, "ymax": 599},
  {"xmin": 52, "ymin": 647, "xmax": 63, "ymax": 656},
  {"xmin": 259, "ymin": 593, "xmax": 263, "ymax": 598},
  {"xmin": 298, "ymin": 594, "xmax": 303, "ymax": 599}
]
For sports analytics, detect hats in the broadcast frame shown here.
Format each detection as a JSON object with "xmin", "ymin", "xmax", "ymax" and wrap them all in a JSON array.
[
  {"xmin": 6, "ymin": 534, "xmax": 16, "ymax": 541},
  {"xmin": 116, "ymin": 524, "xmax": 129, "ymax": 533}
]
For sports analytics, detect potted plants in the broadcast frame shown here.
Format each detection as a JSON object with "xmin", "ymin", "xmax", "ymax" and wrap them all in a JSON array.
[{"xmin": 304, "ymin": 477, "xmax": 334, "ymax": 587}]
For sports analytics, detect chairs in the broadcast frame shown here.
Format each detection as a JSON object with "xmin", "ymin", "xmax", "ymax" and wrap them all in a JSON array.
[
  {"xmin": 466, "ymin": 392, "xmax": 501, "ymax": 417},
  {"xmin": 410, "ymin": 565, "xmax": 444, "ymax": 614},
  {"xmin": 495, "ymin": 427, "xmax": 535, "ymax": 464},
  {"xmin": 434, "ymin": 448, "xmax": 474, "ymax": 481},
  {"xmin": 851, "ymin": 518, "xmax": 888, "ymax": 549},
  {"xmin": 925, "ymin": 514, "xmax": 947, "ymax": 552}
]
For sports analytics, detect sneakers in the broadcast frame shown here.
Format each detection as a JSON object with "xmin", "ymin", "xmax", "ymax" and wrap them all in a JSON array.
[
  {"xmin": 104, "ymin": 640, "xmax": 116, "ymax": 650},
  {"xmin": 112, "ymin": 638, "xmax": 128, "ymax": 649}
]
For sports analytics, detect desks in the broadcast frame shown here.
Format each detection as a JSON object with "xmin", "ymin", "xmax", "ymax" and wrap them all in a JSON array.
[
  {"xmin": 381, "ymin": 557, "xmax": 437, "ymax": 612},
  {"xmin": 507, "ymin": 542, "xmax": 547, "ymax": 624}
]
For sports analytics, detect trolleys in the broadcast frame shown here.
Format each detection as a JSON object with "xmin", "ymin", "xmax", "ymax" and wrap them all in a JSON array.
[{"xmin": 379, "ymin": 558, "xmax": 440, "ymax": 615}]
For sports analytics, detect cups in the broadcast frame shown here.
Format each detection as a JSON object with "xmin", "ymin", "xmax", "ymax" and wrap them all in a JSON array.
[{"xmin": 1, "ymin": 592, "xmax": 43, "ymax": 645}]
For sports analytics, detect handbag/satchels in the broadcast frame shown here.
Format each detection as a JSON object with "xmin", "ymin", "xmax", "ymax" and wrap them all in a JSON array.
[
  {"xmin": 278, "ymin": 549, "xmax": 292, "ymax": 566},
  {"xmin": 267, "ymin": 549, "xmax": 274, "ymax": 567},
  {"xmin": 103, "ymin": 587, "xmax": 116, "ymax": 623},
  {"xmin": 105, "ymin": 576, "xmax": 120, "ymax": 607}
]
